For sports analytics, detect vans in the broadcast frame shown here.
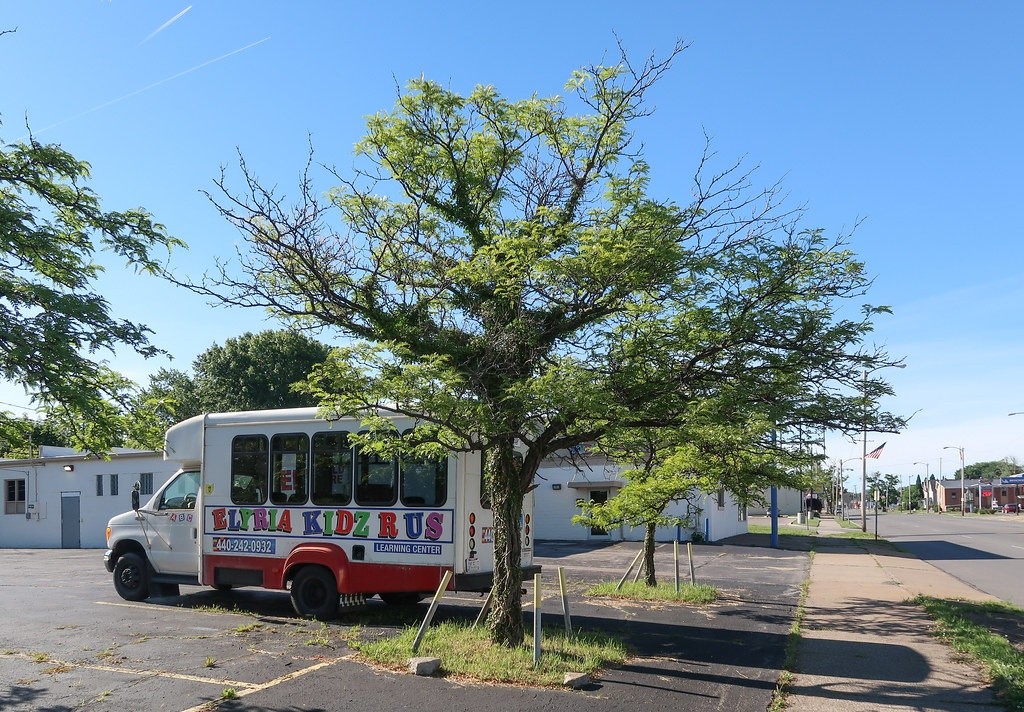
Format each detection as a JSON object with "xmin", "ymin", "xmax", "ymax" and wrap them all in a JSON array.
[{"xmin": 102, "ymin": 402, "xmax": 545, "ymax": 623}]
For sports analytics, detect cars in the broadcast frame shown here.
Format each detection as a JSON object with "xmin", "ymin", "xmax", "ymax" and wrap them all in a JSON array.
[
  {"xmin": 837, "ymin": 508, "xmax": 842, "ymax": 514},
  {"xmin": 993, "ymin": 502, "xmax": 1020, "ymax": 514}
]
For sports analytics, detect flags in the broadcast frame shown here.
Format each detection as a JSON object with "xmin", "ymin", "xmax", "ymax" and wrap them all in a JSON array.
[{"xmin": 864, "ymin": 442, "xmax": 886, "ymax": 459}]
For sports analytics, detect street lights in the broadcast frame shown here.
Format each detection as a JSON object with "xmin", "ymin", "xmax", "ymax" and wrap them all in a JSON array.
[
  {"xmin": 913, "ymin": 462, "xmax": 929, "ymax": 513},
  {"xmin": 834, "ymin": 467, "xmax": 854, "ymax": 516},
  {"xmin": 862, "ymin": 364, "xmax": 907, "ymax": 534},
  {"xmin": 840, "ymin": 457, "xmax": 862, "ymax": 522},
  {"xmin": 943, "ymin": 446, "xmax": 965, "ymax": 516}
]
